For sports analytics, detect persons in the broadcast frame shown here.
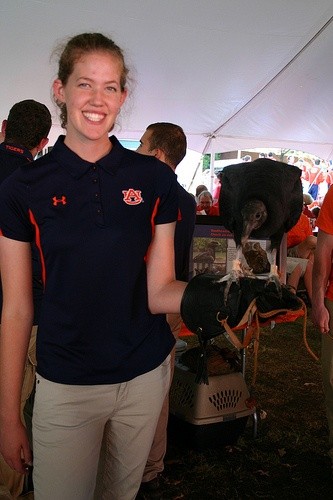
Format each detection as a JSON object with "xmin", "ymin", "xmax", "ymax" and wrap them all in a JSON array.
[
  {"xmin": 286, "ymin": 193, "xmax": 320, "ymax": 299},
  {"xmin": 0, "ymin": 33, "xmax": 302, "ymax": 499},
  {"xmin": 312, "ymin": 185, "xmax": 333, "ymax": 460},
  {"xmin": 140, "ymin": 122, "xmax": 196, "ymax": 491},
  {"xmin": 196, "ymin": 170, "xmax": 222, "ymax": 215},
  {"xmin": 242, "ymin": 150, "xmax": 333, "ymax": 200},
  {"xmin": 0, "ymin": 98, "xmax": 52, "ymax": 499}
]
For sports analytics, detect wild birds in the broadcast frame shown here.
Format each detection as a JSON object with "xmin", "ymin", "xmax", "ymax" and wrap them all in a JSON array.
[{"xmin": 213, "ymin": 157, "xmax": 303, "ymax": 304}]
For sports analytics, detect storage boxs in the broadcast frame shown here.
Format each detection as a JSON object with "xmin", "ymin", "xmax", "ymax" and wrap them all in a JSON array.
[{"xmin": 169, "ymin": 361, "xmax": 257, "ymax": 425}]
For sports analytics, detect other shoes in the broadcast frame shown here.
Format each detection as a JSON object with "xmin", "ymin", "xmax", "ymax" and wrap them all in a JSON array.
[
  {"xmin": 176, "ymin": 339, "xmax": 187, "ymax": 353},
  {"xmin": 139, "ymin": 476, "xmax": 185, "ymax": 500}
]
